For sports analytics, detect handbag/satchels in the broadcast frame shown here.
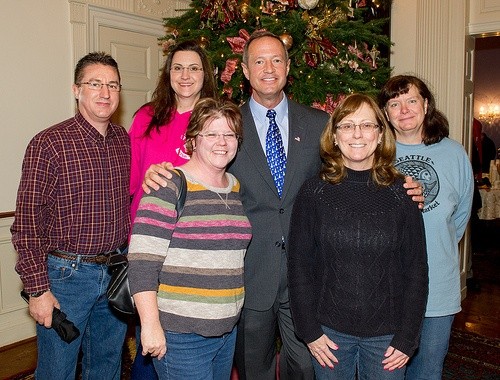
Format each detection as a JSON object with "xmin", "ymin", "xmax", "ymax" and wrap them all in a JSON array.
[{"xmin": 108, "ymin": 169, "xmax": 188, "ymax": 314}]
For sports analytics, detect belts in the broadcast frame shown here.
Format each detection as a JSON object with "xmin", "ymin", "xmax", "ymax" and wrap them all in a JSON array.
[{"xmin": 49, "ymin": 243, "xmax": 128, "ymax": 265}]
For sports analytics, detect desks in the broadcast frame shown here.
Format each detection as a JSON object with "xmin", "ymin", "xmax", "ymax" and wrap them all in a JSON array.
[{"xmin": 477, "ymin": 173, "xmax": 500, "ymax": 220}]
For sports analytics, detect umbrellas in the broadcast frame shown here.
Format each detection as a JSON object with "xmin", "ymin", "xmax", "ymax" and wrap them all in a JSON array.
[{"xmin": 21, "ymin": 289, "xmax": 80, "ymax": 344}]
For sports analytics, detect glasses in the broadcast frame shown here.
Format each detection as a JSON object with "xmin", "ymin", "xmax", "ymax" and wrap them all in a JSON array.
[
  {"xmin": 335, "ymin": 121, "xmax": 380, "ymax": 135},
  {"xmin": 75, "ymin": 81, "xmax": 121, "ymax": 93},
  {"xmin": 198, "ymin": 131, "xmax": 240, "ymax": 142},
  {"xmin": 170, "ymin": 64, "xmax": 204, "ymax": 73}
]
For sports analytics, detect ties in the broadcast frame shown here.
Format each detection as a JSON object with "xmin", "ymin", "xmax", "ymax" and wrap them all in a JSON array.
[{"xmin": 264, "ymin": 110, "xmax": 287, "ymax": 199}]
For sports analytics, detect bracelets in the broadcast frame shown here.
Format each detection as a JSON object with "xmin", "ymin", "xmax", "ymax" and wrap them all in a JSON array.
[{"xmin": 31, "ymin": 290, "xmax": 46, "ymax": 298}]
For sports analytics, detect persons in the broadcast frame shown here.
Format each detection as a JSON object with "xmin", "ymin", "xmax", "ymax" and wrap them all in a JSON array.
[
  {"xmin": 127, "ymin": 98, "xmax": 253, "ymax": 380},
  {"xmin": 11, "ymin": 54, "xmax": 131, "ymax": 380},
  {"xmin": 128, "ymin": 39, "xmax": 216, "ymax": 229},
  {"xmin": 141, "ymin": 30, "xmax": 425, "ymax": 380},
  {"xmin": 375, "ymin": 75, "xmax": 474, "ymax": 380},
  {"xmin": 288, "ymin": 94, "xmax": 429, "ymax": 380}
]
